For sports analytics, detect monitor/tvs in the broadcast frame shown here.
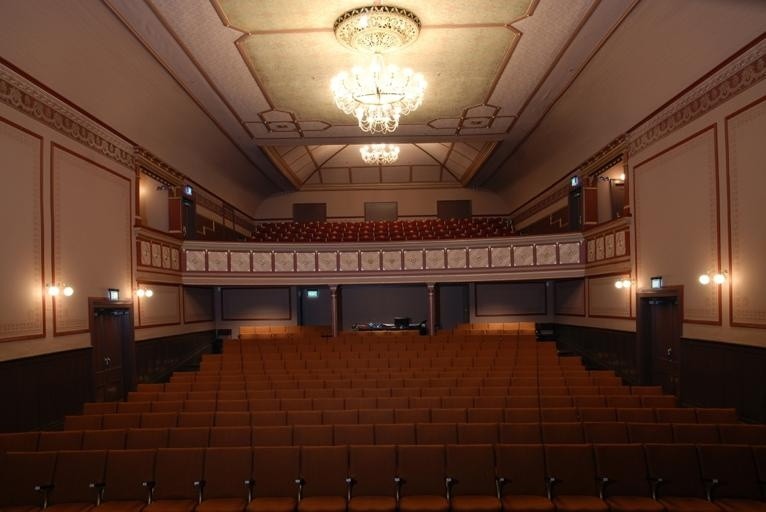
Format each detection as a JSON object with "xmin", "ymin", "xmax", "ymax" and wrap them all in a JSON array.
[{"xmin": 395, "ymin": 319, "xmax": 409, "ymax": 330}]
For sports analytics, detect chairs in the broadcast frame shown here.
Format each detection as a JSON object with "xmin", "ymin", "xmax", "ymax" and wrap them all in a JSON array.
[
  {"xmin": 244, "ymin": 216, "xmax": 521, "ymax": 242},
  {"xmin": 0, "ymin": 321, "xmax": 766, "ymax": 511}
]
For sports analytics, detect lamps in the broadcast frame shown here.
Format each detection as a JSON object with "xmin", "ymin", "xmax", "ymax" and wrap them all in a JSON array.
[
  {"xmin": 331, "ymin": 6, "xmax": 427, "ymax": 135},
  {"xmin": 359, "ymin": 143, "xmax": 401, "ymax": 167}
]
